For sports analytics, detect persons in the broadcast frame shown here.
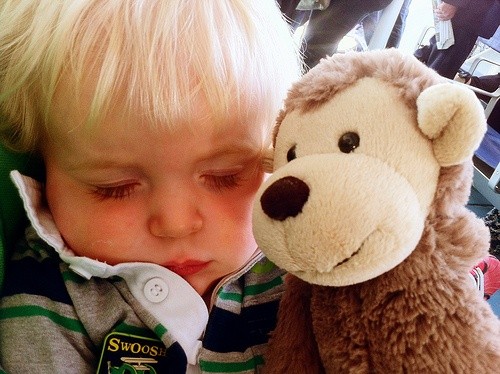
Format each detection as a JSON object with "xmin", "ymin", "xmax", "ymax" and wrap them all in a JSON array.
[
  {"xmin": 280, "ymin": 0, "xmax": 395, "ymax": 79},
  {"xmin": 409, "ymin": 0, "xmax": 499, "ymax": 80},
  {"xmin": 2, "ymin": 0, "xmax": 298, "ymax": 374}
]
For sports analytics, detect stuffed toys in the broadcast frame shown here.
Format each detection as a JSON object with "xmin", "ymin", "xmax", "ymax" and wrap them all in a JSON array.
[{"xmin": 251, "ymin": 46, "xmax": 500, "ymax": 374}]
